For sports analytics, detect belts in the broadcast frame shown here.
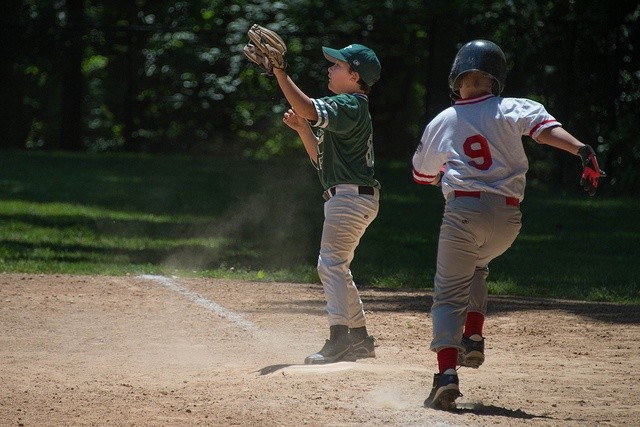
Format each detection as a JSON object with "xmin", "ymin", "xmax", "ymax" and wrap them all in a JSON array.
[
  {"xmin": 329, "ymin": 184, "xmax": 375, "ymax": 196},
  {"xmin": 453, "ymin": 189, "xmax": 520, "ymax": 207}
]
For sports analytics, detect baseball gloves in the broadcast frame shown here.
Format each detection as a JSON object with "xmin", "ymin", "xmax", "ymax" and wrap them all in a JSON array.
[{"xmin": 243, "ymin": 24, "xmax": 289, "ymax": 77}]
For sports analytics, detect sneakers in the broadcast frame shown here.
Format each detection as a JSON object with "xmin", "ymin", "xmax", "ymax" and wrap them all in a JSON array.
[
  {"xmin": 348, "ymin": 335, "xmax": 377, "ymax": 358},
  {"xmin": 424, "ymin": 367, "xmax": 460, "ymax": 408},
  {"xmin": 305, "ymin": 338, "xmax": 354, "ymax": 362},
  {"xmin": 457, "ymin": 332, "xmax": 484, "ymax": 367}
]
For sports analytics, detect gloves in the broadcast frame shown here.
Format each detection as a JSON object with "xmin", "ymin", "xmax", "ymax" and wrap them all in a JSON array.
[{"xmin": 578, "ymin": 145, "xmax": 606, "ymax": 197}]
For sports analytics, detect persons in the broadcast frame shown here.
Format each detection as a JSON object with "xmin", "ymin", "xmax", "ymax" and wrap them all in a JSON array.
[
  {"xmin": 242, "ymin": 25, "xmax": 382, "ymax": 363},
  {"xmin": 411, "ymin": 40, "xmax": 607, "ymax": 412}
]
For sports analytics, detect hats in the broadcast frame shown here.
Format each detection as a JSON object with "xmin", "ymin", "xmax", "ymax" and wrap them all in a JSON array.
[{"xmin": 320, "ymin": 44, "xmax": 382, "ymax": 87}]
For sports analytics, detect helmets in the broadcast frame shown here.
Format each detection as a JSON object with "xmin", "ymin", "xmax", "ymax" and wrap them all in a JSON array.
[{"xmin": 448, "ymin": 39, "xmax": 508, "ymax": 95}]
